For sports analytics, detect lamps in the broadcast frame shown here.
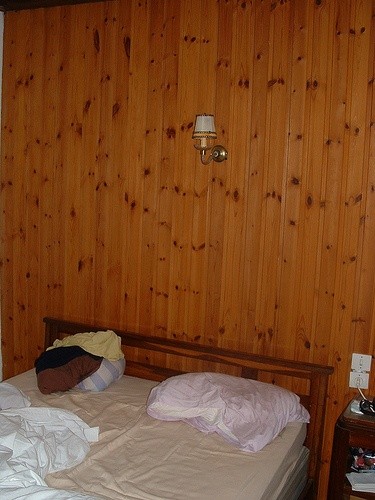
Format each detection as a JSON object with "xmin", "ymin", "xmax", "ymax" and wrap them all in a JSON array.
[{"xmin": 192, "ymin": 113, "xmax": 227, "ymax": 165}]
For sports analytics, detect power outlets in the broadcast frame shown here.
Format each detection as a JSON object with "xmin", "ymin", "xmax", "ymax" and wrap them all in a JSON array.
[{"xmin": 349, "ymin": 372, "xmax": 369, "ymax": 389}]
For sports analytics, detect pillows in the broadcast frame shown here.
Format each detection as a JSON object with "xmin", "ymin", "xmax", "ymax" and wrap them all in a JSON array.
[{"xmin": 146, "ymin": 372, "xmax": 311, "ymax": 453}]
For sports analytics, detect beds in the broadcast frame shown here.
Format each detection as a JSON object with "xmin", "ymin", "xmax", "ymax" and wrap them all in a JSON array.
[{"xmin": 0, "ymin": 317, "xmax": 335, "ymax": 500}]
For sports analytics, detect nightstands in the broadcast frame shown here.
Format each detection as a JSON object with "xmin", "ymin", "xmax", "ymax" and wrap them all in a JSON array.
[{"xmin": 327, "ymin": 393, "xmax": 375, "ymax": 500}]
